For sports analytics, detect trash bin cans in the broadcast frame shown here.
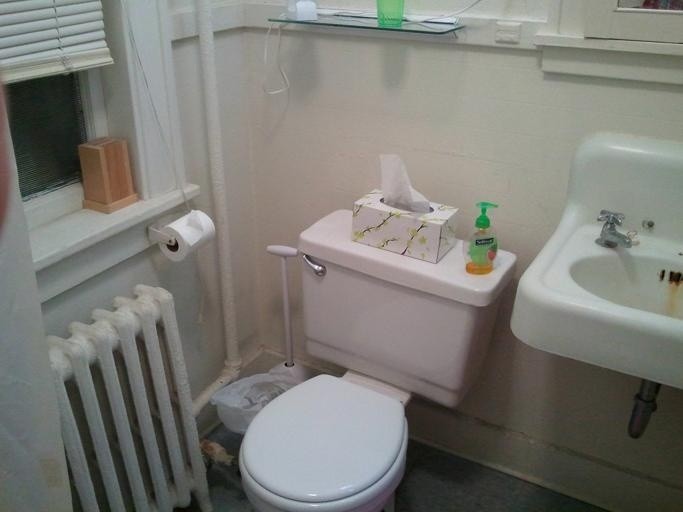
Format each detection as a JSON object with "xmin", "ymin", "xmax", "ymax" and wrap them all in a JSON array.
[{"xmin": 212, "ymin": 373, "xmax": 302, "ymax": 448}]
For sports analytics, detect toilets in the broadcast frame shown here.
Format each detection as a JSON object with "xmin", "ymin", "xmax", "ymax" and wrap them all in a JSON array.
[{"xmin": 238, "ymin": 209, "xmax": 516, "ymax": 512}]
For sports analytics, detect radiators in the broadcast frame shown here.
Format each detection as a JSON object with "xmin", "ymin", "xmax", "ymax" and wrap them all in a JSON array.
[{"xmin": 46, "ymin": 284, "xmax": 214, "ymax": 512}]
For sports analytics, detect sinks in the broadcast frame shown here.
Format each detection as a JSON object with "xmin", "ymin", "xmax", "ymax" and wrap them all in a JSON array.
[{"xmin": 510, "ymin": 134, "xmax": 683, "ymax": 391}]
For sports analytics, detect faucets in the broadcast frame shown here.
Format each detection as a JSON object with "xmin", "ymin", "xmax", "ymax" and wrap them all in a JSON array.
[{"xmin": 595, "ymin": 210, "xmax": 633, "ymax": 249}]
